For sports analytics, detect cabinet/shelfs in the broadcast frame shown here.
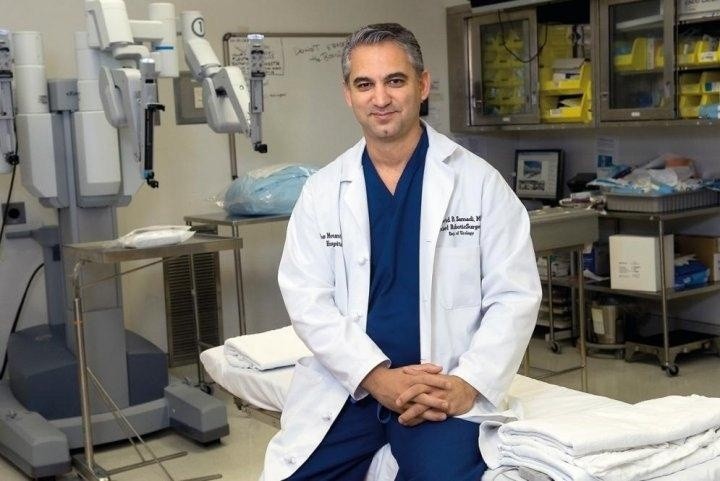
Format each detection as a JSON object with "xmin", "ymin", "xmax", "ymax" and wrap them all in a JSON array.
[
  {"xmin": 445, "ymin": 0, "xmax": 720, "ymax": 136},
  {"xmin": 522, "ymin": 207, "xmax": 720, "ymax": 394}
]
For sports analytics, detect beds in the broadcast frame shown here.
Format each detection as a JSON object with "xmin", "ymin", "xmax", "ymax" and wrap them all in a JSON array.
[{"xmin": 198, "ymin": 321, "xmax": 720, "ymax": 480}]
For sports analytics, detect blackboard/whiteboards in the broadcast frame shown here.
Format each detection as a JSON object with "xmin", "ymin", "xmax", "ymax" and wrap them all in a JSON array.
[{"xmin": 222, "ymin": 32, "xmax": 365, "ymax": 181}]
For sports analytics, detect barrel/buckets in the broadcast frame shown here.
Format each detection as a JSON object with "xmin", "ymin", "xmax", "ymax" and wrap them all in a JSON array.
[{"xmin": 591, "ymin": 302, "xmax": 624, "ymax": 344}]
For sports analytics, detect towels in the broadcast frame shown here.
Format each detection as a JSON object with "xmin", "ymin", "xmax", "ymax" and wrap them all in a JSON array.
[
  {"xmin": 224, "ymin": 324, "xmax": 314, "ymax": 372},
  {"xmin": 495, "ymin": 392, "xmax": 719, "ymax": 480}
]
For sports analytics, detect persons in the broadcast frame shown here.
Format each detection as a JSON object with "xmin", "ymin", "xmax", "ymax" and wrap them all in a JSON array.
[{"xmin": 258, "ymin": 20, "xmax": 544, "ymax": 481}]
